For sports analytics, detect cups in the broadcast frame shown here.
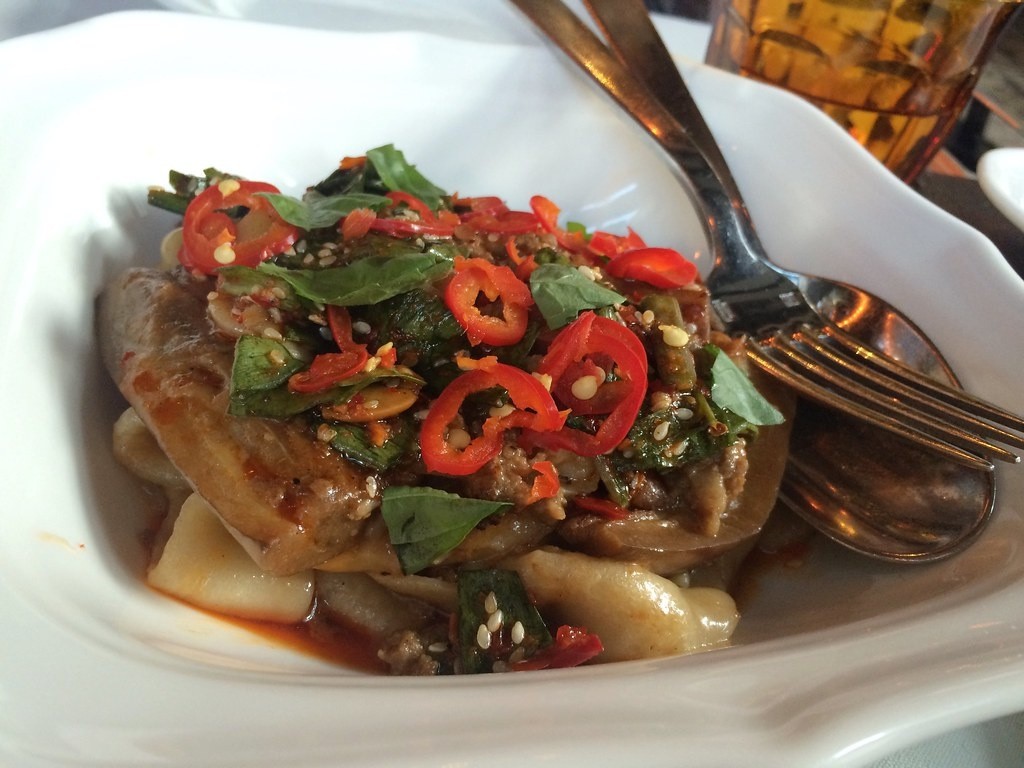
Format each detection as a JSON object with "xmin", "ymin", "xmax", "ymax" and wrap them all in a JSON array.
[{"xmin": 712, "ymin": 0, "xmax": 1015, "ymax": 186}]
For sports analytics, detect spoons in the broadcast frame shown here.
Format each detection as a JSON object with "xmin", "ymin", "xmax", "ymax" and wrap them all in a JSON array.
[{"xmin": 588, "ymin": 0, "xmax": 1000, "ymax": 571}]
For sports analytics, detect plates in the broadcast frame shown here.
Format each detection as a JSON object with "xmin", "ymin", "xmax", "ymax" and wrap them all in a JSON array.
[{"xmin": 0, "ymin": 12, "xmax": 1021, "ymax": 768}]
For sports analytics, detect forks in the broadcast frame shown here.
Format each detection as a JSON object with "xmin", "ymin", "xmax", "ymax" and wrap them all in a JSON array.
[{"xmin": 521, "ymin": 0, "xmax": 1021, "ymax": 489}]
